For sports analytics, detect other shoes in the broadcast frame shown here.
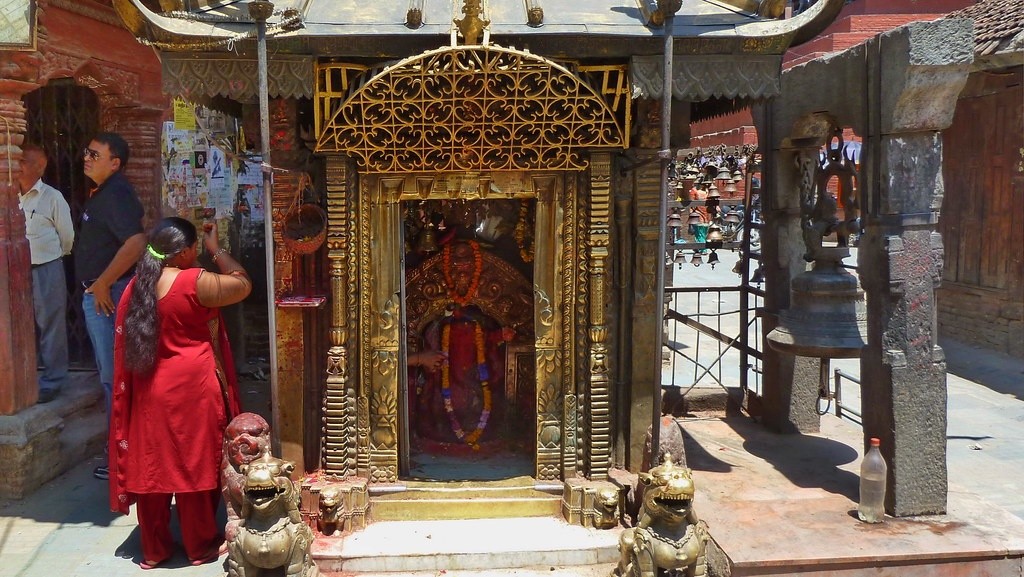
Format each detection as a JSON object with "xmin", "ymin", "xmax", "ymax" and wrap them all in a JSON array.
[
  {"xmin": 140, "ymin": 538, "xmax": 179, "ymax": 569},
  {"xmin": 36, "ymin": 386, "xmax": 60, "ymax": 403},
  {"xmin": 190, "ymin": 535, "xmax": 228, "ymax": 567}
]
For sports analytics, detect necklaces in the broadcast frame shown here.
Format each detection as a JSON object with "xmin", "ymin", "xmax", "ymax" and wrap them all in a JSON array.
[
  {"xmin": 442, "ymin": 314, "xmax": 491, "ymax": 442},
  {"xmin": 444, "ymin": 239, "xmax": 481, "ymax": 305},
  {"xmin": 517, "ymin": 198, "xmax": 535, "ymax": 262}
]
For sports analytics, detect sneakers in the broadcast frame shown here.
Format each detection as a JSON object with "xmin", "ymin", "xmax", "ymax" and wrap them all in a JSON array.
[{"xmin": 93, "ymin": 466, "xmax": 110, "ymax": 480}]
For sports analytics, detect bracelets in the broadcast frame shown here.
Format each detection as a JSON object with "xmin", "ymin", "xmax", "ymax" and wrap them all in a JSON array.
[
  {"xmin": 212, "ymin": 248, "xmax": 226, "ymax": 263},
  {"xmin": 418, "ymin": 352, "xmax": 422, "ymax": 366}
]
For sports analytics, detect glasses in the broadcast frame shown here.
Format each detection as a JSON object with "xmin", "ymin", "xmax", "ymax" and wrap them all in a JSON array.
[{"xmin": 84, "ymin": 148, "xmax": 114, "ymax": 161}]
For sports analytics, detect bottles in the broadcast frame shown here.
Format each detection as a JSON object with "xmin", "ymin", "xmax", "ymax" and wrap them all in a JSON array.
[{"xmin": 857, "ymin": 437, "xmax": 888, "ymax": 523}]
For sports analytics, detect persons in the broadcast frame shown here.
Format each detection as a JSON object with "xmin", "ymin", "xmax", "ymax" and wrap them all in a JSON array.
[
  {"xmin": 407, "ymin": 345, "xmax": 445, "ymax": 372},
  {"xmin": 109, "ymin": 217, "xmax": 252, "ymax": 569},
  {"xmin": 77, "ymin": 133, "xmax": 146, "ymax": 478},
  {"xmin": 19, "ymin": 146, "xmax": 74, "ymax": 402},
  {"xmin": 689, "ymin": 173, "xmax": 712, "ymax": 255}
]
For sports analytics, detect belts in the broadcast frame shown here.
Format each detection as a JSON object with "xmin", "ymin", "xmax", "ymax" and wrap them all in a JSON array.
[{"xmin": 81, "ymin": 281, "xmax": 95, "ymax": 288}]
